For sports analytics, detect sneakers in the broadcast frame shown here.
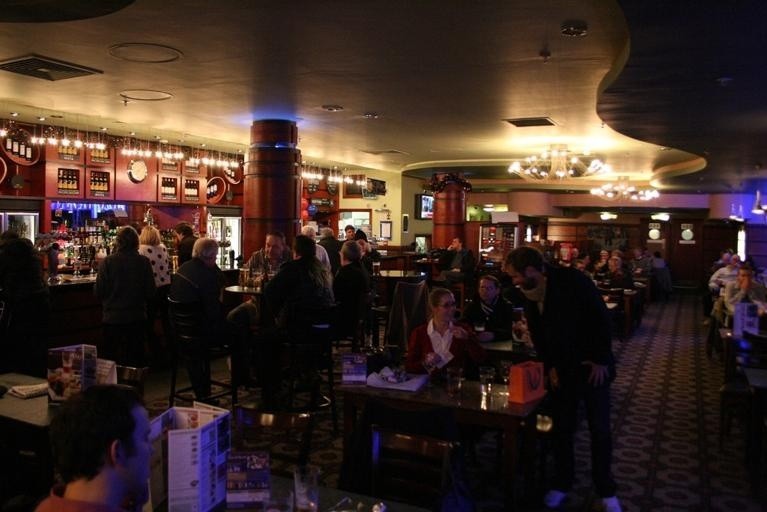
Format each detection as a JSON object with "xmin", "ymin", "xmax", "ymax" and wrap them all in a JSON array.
[
  {"xmin": 603, "ymin": 496, "xmax": 621, "ymax": 512},
  {"xmin": 546, "ymin": 489, "xmax": 567, "ymax": 506}
]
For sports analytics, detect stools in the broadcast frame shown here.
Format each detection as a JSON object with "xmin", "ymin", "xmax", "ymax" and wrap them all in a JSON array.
[{"xmin": 452, "ymin": 282, "xmax": 465, "ymax": 318}]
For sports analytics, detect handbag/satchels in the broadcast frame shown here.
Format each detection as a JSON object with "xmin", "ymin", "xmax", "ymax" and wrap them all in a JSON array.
[{"xmin": 509, "ymin": 362, "xmax": 545, "ymax": 404}]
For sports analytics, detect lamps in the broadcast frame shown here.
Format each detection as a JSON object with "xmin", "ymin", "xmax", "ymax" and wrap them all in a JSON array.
[
  {"xmin": 508, "ymin": 142, "xmax": 606, "ymax": 188},
  {"xmin": 589, "ymin": 175, "xmax": 660, "ymax": 202}
]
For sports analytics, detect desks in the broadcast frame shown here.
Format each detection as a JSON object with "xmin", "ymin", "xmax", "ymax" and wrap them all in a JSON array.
[
  {"xmin": 0, "ymin": 369, "xmax": 54, "ymax": 482},
  {"xmin": 215, "ymin": 467, "xmax": 429, "ymax": 510},
  {"xmin": 602, "ymin": 276, "xmax": 651, "ymax": 335},
  {"xmin": 340, "ymin": 374, "xmax": 549, "ymax": 504},
  {"xmin": 377, "ymin": 269, "xmax": 425, "ymax": 304}
]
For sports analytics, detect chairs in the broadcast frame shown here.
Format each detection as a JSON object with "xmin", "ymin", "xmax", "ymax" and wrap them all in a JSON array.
[
  {"xmin": 385, "ymin": 278, "xmax": 428, "ymax": 344},
  {"xmin": 716, "ymin": 324, "xmax": 766, "ymax": 446},
  {"xmin": 164, "ymin": 297, "xmax": 338, "ymax": 441}
]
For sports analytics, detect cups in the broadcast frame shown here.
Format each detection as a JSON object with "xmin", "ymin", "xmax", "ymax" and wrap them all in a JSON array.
[
  {"xmin": 238, "ymin": 268, "xmax": 249, "ymax": 286},
  {"xmin": 251, "ymin": 270, "xmax": 262, "ymax": 287},
  {"xmin": 295, "ymin": 463, "xmax": 318, "ymax": 509},
  {"xmin": 448, "ymin": 369, "xmax": 463, "ymax": 396},
  {"xmin": 478, "ymin": 366, "xmax": 496, "ymax": 394}
]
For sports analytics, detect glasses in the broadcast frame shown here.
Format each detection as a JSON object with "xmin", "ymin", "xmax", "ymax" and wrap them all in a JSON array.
[
  {"xmin": 442, "ymin": 301, "xmax": 457, "ymax": 307},
  {"xmin": 480, "ymin": 286, "xmax": 493, "ymax": 290}
]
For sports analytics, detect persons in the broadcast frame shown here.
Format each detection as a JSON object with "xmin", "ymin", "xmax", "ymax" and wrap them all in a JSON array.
[
  {"xmin": 439, "ymin": 236, "xmax": 477, "ymax": 284},
  {"xmin": 239, "ymin": 225, "xmax": 384, "ymax": 411},
  {"xmin": 463, "ymin": 273, "xmax": 513, "ymax": 382},
  {"xmin": 545, "ymin": 245, "xmax": 668, "ymax": 323},
  {"xmin": 502, "ymin": 246, "xmax": 627, "ymax": 511},
  {"xmin": 95, "ymin": 219, "xmax": 230, "ymax": 408},
  {"xmin": 403, "ymin": 284, "xmax": 486, "ymax": 460},
  {"xmin": 1, "ymin": 217, "xmax": 51, "ymax": 380},
  {"xmin": 700, "ymin": 246, "xmax": 767, "ymax": 397},
  {"xmin": 502, "ymin": 240, "xmax": 627, "ymax": 511},
  {"xmin": 488, "ymin": 241, "xmax": 501, "ymax": 257},
  {"xmin": 31, "ymin": 383, "xmax": 152, "ymax": 512}
]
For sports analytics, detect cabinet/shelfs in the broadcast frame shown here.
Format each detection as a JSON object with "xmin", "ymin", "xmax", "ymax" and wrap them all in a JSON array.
[
  {"xmin": 156, "ymin": 144, "xmax": 208, "ymax": 208},
  {"xmin": 31, "ymin": 130, "xmax": 116, "ymax": 201}
]
